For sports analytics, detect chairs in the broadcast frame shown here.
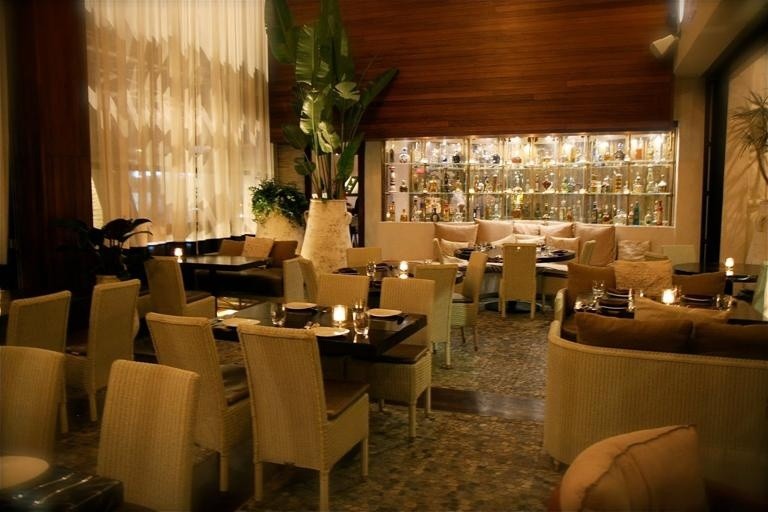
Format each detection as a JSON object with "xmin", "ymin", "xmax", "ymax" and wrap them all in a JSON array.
[{"xmin": 498, "ymin": 243, "xmax": 537, "ymax": 321}]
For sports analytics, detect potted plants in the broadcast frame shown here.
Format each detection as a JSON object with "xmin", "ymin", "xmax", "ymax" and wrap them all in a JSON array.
[{"xmin": 265, "ymin": 0, "xmax": 398, "ymax": 276}]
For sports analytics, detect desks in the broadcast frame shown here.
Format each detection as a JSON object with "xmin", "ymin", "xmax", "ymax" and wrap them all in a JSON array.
[{"xmin": 672, "ymin": 262, "xmax": 760, "ymax": 295}]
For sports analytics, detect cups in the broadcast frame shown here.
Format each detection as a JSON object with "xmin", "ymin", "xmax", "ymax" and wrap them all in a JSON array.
[
  {"xmin": 591, "ymin": 279, "xmax": 606, "ymax": 297},
  {"xmin": 480, "ymin": 241, "xmax": 488, "ymax": 252},
  {"xmin": 716, "ymin": 294, "xmax": 730, "ymax": 311},
  {"xmin": 353, "ymin": 307, "xmax": 371, "ymax": 335},
  {"xmin": 540, "ymin": 245, "xmax": 550, "ymax": 257},
  {"xmin": 366, "ymin": 257, "xmax": 377, "ymax": 277},
  {"xmin": 270, "ymin": 304, "xmax": 287, "ymax": 326},
  {"xmin": 351, "ymin": 296, "xmax": 366, "ymax": 314},
  {"xmin": 400, "ymin": 179, "xmax": 408, "ymax": 192},
  {"xmin": 627, "ymin": 287, "xmax": 644, "ymax": 312}
]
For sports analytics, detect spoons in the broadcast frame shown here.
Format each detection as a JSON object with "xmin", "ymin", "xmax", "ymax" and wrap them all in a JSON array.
[
  {"xmin": 30, "ymin": 476, "xmax": 92, "ymax": 506},
  {"xmin": 308, "ymin": 306, "xmax": 330, "ymax": 313},
  {"xmin": 12, "ymin": 473, "xmax": 73, "ymax": 500}
]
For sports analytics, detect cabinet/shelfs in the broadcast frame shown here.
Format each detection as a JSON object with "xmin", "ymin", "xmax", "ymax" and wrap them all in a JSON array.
[{"xmin": 382, "ymin": 128, "xmax": 679, "ymax": 227}]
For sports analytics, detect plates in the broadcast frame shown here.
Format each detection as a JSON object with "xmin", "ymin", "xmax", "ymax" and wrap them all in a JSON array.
[
  {"xmin": 311, "ymin": 327, "xmax": 350, "ymax": 337},
  {"xmin": 365, "ymin": 308, "xmax": 402, "ymax": 318},
  {"xmin": 282, "ymin": 302, "xmax": 318, "ymax": 310},
  {"xmin": 0, "ymin": 455, "xmax": 49, "ymax": 490},
  {"xmin": 223, "ymin": 318, "xmax": 261, "ymax": 327}
]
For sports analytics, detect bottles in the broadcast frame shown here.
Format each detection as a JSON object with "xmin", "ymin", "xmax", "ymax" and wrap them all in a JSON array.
[
  {"xmin": 633, "ymin": 201, "xmax": 640, "ymax": 225},
  {"xmin": 591, "ymin": 202, "xmax": 598, "ymax": 223},
  {"xmin": 656, "ymin": 200, "xmax": 663, "ymax": 226},
  {"xmin": 535, "ymin": 202, "xmax": 541, "ymax": 219},
  {"xmin": 389, "ymin": 201, "xmax": 395, "ymax": 222},
  {"xmin": 542, "ymin": 203, "xmax": 549, "ymax": 220},
  {"xmin": 401, "ymin": 209, "xmax": 407, "ymax": 221},
  {"xmin": 412, "ymin": 200, "xmax": 417, "ymax": 221},
  {"xmin": 567, "ymin": 207, "xmax": 572, "ymax": 222},
  {"xmin": 492, "ymin": 174, "xmax": 498, "ymax": 192},
  {"xmin": 657, "ymin": 174, "xmax": 667, "ymax": 193},
  {"xmin": 431, "ymin": 208, "xmax": 439, "ymax": 222},
  {"xmin": 645, "ymin": 207, "xmax": 652, "ymax": 225}
]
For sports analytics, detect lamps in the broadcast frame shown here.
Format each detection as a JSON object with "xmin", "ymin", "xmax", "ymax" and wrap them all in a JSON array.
[{"xmin": 651, "ymin": 34, "xmax": 678, "ymax": 59}]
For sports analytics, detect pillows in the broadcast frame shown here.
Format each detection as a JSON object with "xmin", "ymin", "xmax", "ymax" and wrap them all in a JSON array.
[
  {"xmin": 672, "ymin": 271, "xmax": 726, "ymax": 300},
  {"xmin": 567, "ymin": 262, "xmax": 616, "ymax": 314},
  {"xmin": 433, "ymin": 218, "xmax": 651, "ymax": 262},
  {"xmin": 558, "ymin": 423, "xmax": 708, "ymax": 512}
]
